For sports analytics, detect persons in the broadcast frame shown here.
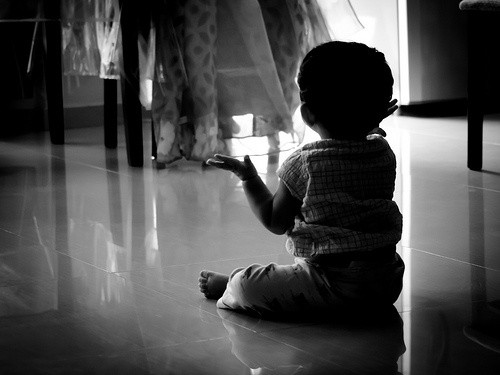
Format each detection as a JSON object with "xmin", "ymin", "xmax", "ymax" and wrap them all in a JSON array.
[{"xmin": 198, "ymin": 40, "xmax": 403, "ymax": 317}]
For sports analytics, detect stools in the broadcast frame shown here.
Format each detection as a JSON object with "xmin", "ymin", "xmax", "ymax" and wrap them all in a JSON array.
[{"xmin": 459, "ymin": 0, "xmax": 500, "ymax": 170}]
